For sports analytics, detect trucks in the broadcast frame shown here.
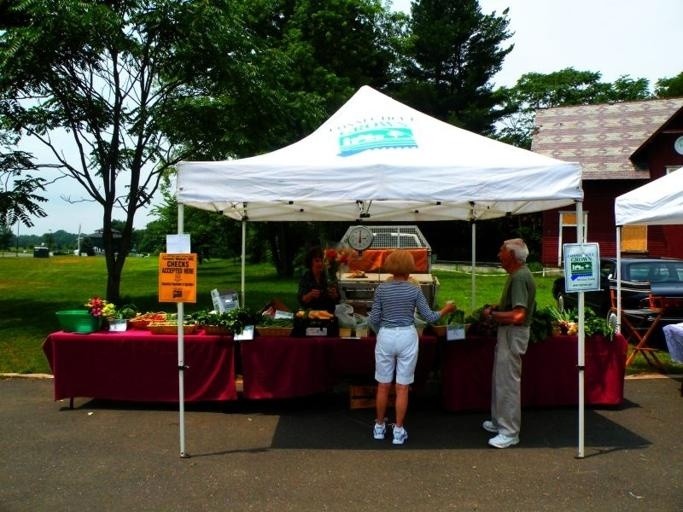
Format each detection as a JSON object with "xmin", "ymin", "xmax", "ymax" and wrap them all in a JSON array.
[{"xmin": 326, "ymin": 223, "xmax": 439, "ymax": 312}]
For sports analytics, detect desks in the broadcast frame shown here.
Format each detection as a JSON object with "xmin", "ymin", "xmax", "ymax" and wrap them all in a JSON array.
[{"xmin": 662, "ymin": 322, "xmax": 683, "ymax": 365}]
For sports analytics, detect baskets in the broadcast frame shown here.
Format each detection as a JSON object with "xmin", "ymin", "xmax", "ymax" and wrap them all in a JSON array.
[
  {"xmin": 256, "ymin": 324, "xmax": 294, "ymax": 337},
  {"xmin": 430, "ymin": 323, "xmax": 471, "ymax": 336},
  {"xmin": 129, "ymin": 313, "xmax": 199, "ymax": 334},
  {"xmin": 204, "ymin": 323, "xmax": 232, "ymax": 335}
]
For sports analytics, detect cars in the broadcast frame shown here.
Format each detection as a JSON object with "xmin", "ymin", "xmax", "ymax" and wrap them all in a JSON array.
[{"xmin": 552, "ymin": 256, "xmax": 682, "ymax": 342}]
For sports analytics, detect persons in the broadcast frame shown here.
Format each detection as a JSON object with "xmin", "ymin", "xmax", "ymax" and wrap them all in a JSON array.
[
  {"xmin": 365, "ymin": 249, "xmax": 456, "ymax": 444},
  {"xmin": 298, "ymin": 246, "xmax": 341, "ymax": 313},
  {"xmin": 481, "ymin": 238, "xmax": 536, "ymax": 449}
]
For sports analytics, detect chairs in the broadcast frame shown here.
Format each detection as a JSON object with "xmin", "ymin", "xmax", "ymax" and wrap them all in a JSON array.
[{"xmin": 609, "ymin": 278, "xmax": 670, "ymax": 374}]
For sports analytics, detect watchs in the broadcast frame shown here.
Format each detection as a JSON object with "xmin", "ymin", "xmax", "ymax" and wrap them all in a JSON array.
[{"xmin": 488, "ymin": 310, "xmax": 494, "ymax": 321}]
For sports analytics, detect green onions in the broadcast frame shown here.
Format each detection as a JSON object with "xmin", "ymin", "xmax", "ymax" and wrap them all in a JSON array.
[{"xmin": 543, "ymin": 304, "xmax": 569, "ymax": 330}]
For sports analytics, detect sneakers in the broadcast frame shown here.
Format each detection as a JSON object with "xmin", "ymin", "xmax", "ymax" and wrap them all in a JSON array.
[
  {"xmin": 488, "ymin": 434, "xmax": 519, "ymax": 448},
  {"xmin": 484, "ymin": 421, "xmax": 497, "ymax": 433},
  {"xmin": 373, "ymin": 423, "xmax": 386, "ymax": 439},
  {"xmin": 392, "ymin": 427, "xmax": 408, "ymax": 445}
]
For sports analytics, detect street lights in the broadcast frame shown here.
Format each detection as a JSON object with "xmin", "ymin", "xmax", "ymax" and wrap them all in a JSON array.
[
  {"xmin": 75, "ymin": 224, "xmax": 83, "ymax": 256},
  {"xmin": 6, "ymin": 217, "xmax": 18, "ymax": 256},
  {"xmin": 47, "ymin": 228, "xmax": 52, "ymax": 251}
]
